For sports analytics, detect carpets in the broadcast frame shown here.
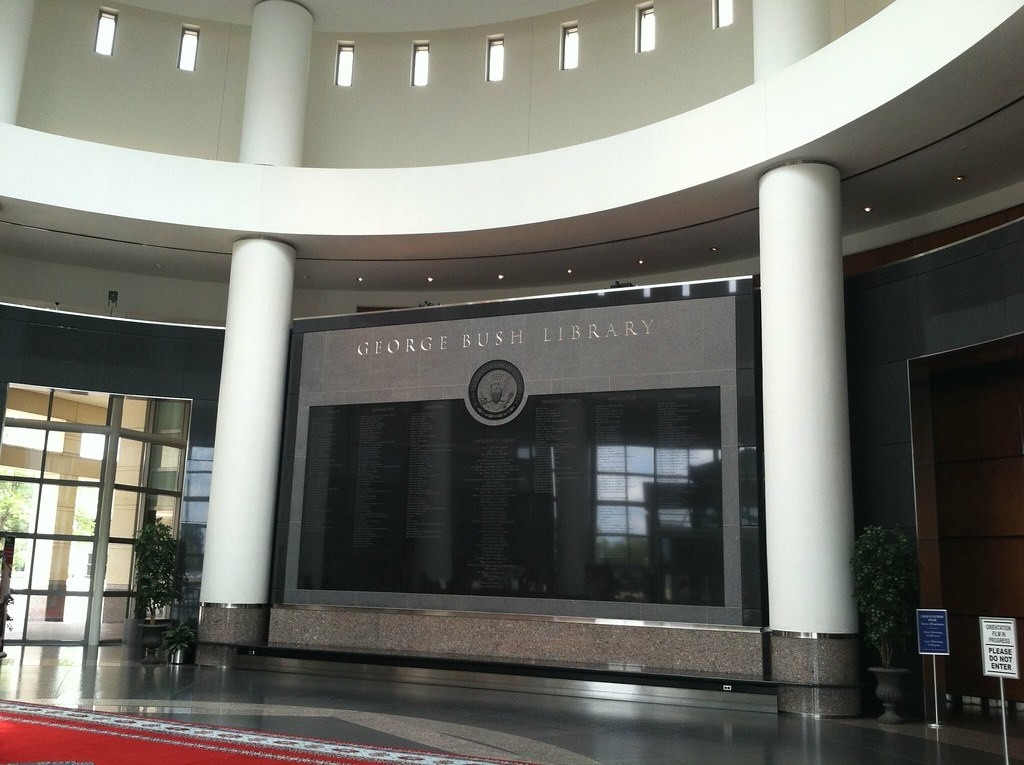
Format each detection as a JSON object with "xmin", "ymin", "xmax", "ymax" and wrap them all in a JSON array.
[{"xmin": 1, "ymin": 700, "xmax": 534, "ymax": 765}]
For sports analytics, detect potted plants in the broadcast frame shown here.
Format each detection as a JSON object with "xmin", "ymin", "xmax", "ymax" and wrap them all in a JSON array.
[
  {"xmin": 161, "ymin": 623, "xmax": 195, "ymax": 664},
  {"xmin": 132, "ymin": 517, "xmax": 189, "ymax": 665},
  {"xmin": 849, "ymin": 526, "xmax": 920, "ymax": 724}
]
